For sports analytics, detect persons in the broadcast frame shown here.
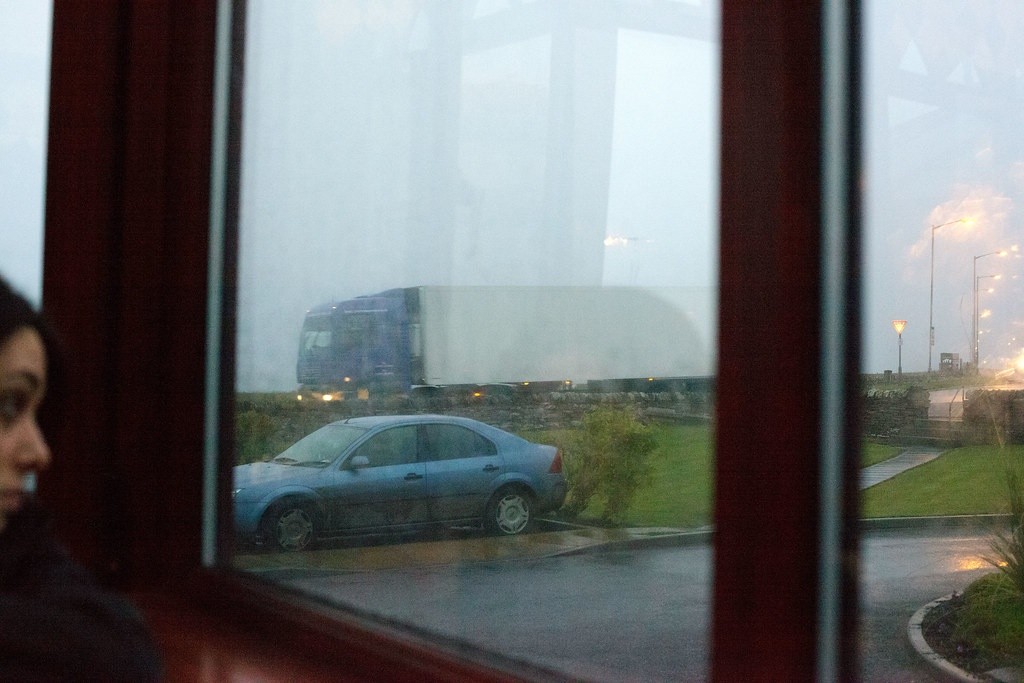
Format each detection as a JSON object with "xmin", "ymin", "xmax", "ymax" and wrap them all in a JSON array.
[{"xmin": 2, "ymin": 277, "xmax": 170, "ymax": 681}]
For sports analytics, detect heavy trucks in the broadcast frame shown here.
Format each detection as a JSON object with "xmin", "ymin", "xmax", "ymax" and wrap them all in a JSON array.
[{"xmin": 294, "ymin": 277, "xmax": 716, "ymax": 405}]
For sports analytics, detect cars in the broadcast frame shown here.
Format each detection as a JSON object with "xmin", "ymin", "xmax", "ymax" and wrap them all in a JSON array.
[{"xmin": 228, "ymin": 414, "xmax": 570, "ymax": 553}]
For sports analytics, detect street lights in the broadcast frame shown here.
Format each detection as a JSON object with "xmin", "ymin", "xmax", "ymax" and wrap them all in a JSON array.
[
  {"xmin": 973, "ymin": 244, "xmax": 1020, "ymax": 369},
  {"xmin": 927, "ymin": 216, "xmax": 973, "ymax": 371},
  {"xmin": 893, "ymin": 316, "xmax": 907, "ymax": 376}
]
